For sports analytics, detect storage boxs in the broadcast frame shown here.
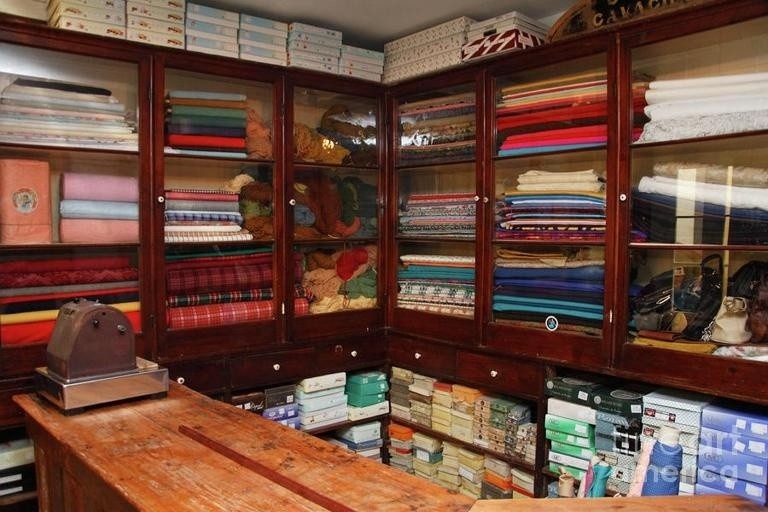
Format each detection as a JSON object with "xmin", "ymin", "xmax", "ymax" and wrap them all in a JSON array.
[{"xmin": 1, "ymin": 437, "xmax": 38, "ymax": 499}]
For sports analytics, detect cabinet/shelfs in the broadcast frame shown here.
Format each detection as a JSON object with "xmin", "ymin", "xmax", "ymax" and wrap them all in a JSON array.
[
  {"xmin": 0, "ymin": 15, "xmax": 386, "ymax": 512},
  {"xmin": 386, "ymin": 0, "xmax": 767, "ymax": 499}
]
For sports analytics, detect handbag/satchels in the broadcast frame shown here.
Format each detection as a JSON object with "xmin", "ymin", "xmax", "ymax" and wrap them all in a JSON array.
[{"xmin": 628, "ymin": 261, "xmax": 767, "ymax": 345}]
